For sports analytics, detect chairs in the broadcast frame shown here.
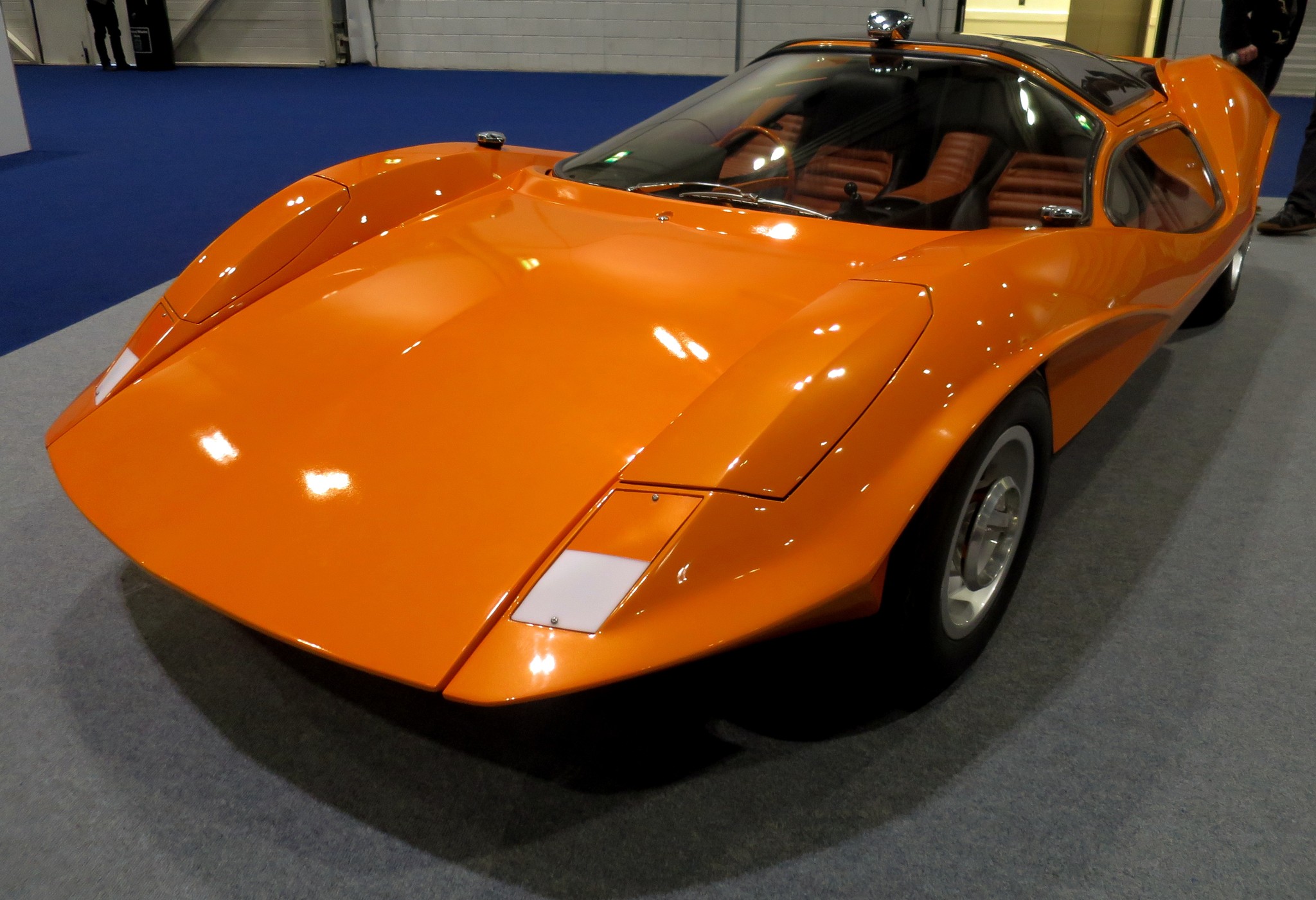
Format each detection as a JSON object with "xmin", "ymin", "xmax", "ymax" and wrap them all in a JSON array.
[
  {"xmin": 950, "ymin": 131, "xmax": 1093, "ymax": 220},
  {"xmin": 717, "ymin": 115, "xmax": 903, "ymax": 219}
]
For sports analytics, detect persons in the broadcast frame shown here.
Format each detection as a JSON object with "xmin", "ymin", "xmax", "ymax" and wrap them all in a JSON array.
[
  {"xmin": 1254, "ymin": 95, "xmax": 1316, "ymax": 237},
  {"xmin": 1217, "ymin": 0, "xmax": 1309, "ymax": 104},
  {"xmin": 86, "ymin": 0, "xmax": 135, "ymax": 70}
]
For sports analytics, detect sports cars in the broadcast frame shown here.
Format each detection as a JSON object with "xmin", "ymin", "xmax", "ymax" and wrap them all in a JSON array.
[{"xmin": 47, "ymin": 7, "xmax": 1282, "ymax": 713}]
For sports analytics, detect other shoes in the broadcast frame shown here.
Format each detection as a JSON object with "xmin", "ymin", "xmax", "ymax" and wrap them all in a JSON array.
[
  {"xmin": 1257, "ymin": 204, "xmax": 1316, "ymax": 234},
  {"xmin": 117, "ymin": 64, "xmax": 136, "ymax": 70},
  {"xmin": 1256, "ymin": 205, "xmax": 1263, "ymax": 215},
  {"xmin": 103, "ymin": 65, "xmax": 117, "ymax": 71}
]
